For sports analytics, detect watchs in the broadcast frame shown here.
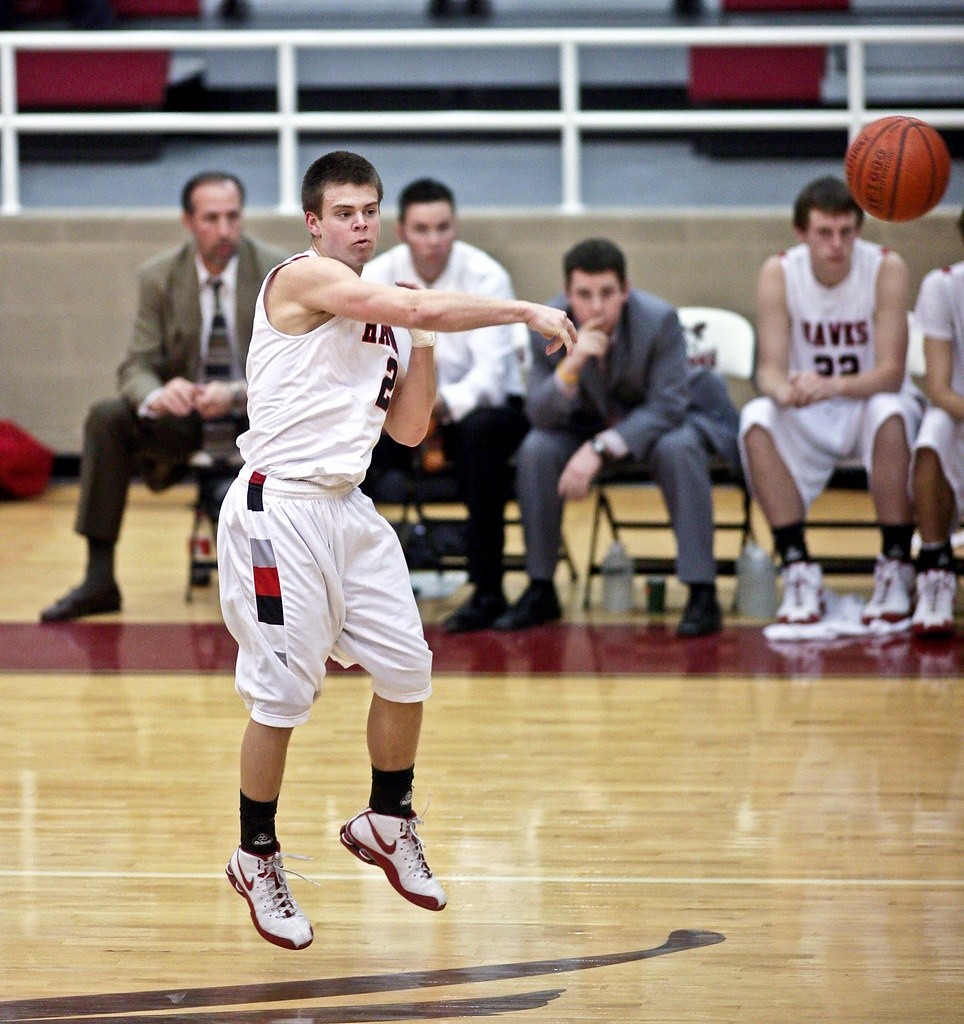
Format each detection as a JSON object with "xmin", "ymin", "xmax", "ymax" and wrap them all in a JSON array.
[{"xmin": 590, "ymin": 433, "xmax": 616, "ymax": 467}]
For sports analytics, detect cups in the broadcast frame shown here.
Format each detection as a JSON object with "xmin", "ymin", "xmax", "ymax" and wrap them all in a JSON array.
[{"xmin": 646, "ymin": 581, "xmax": 666, "ymax": 613}]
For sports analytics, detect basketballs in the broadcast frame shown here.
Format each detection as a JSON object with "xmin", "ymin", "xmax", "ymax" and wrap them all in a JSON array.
[{"xmin": 844, "ymin": 115, "xmax": 951, "ymax": 224}]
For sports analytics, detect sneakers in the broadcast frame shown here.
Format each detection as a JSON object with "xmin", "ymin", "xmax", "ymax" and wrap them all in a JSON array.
[
  {"xmin": 339, "ymin": 791, "xmax": 447, "ymax": 913},
  {"xmin": 861, "ymin": 551, "xmax": 915, "ymax": 623},
  {"xmin": 773, "ymin": 561, "xmax": 828, "ymax": 623},
  {"xmin": 223, "ymin": 844, "xmax": 322, "ymax": 950},
  {"xmin": 911, "ymin": 570, "xmax": 958, "ymax": 631}
]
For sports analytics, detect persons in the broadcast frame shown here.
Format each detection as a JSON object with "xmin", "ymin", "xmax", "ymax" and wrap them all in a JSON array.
[
  {"xmin": 359, "ymin": 180, "xmax": 532, "ymax": 637},
  {"xmin": 911, "ymin": 210, "xmax": 964, "ymax": 638},
  {"xmin": 40, "ymin": 172, "xmax": 290, "ymax": 626},
  {"xmin": 218, "ymin": 150, "xmax": 576, "ymax": 954},
  {"xmin": 494, "ymin": 237, "xmax": 740, "ymax": 637},
  {"xmin": 736, "ymin": 173, "xmax": 905, "ymax": 641}
]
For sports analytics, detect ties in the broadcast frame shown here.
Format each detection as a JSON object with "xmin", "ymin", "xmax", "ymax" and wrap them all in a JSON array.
[{"xmin": 203, "ymin": 281, "xmax": 235, "ymax": 461}]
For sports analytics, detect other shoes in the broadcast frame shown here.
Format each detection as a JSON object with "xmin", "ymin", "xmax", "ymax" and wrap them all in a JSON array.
[
  {"xmin": 41, "ymin": 578, "xmax": 124, "ymax": 622},
  {"xmin": 677, "ymin": 595, "xmax": 724, "ymax": 636},
  {"xmin": 494, "ymin": 591, "xmax": 563, "ymax": 632},
  {"xmin": 438, "ymin": 592, "xmax": 509, "ymax": 634}
]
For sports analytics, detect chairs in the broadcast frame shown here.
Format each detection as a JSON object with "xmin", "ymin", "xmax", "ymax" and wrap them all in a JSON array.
[
  {"xmin": 397, "ymin": 498, "xmax": 575, "ymax": 604},
  {"xmin": 184, "ymin": 483, "xmax": 231, "ymax": 599},
  {"xmin": 587, "ymin": 305, "xmax": 752, "ymax": 608},
  {"xmin": 765, "ymin": 462, "xmax": 885, "ymax": 580}
]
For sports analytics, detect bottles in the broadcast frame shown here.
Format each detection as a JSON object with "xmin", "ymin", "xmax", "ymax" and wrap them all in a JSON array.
[
  {"xmin": 603, "ymin": 544, "xmax": 632, "ymax": 614},
  {"xmin": 737, "ymin": 542, "xmax": 774, "ymax": 616},
  {"xmin": 193, "ymin": 539, "xmax": 214, "ymax": 599}
]
